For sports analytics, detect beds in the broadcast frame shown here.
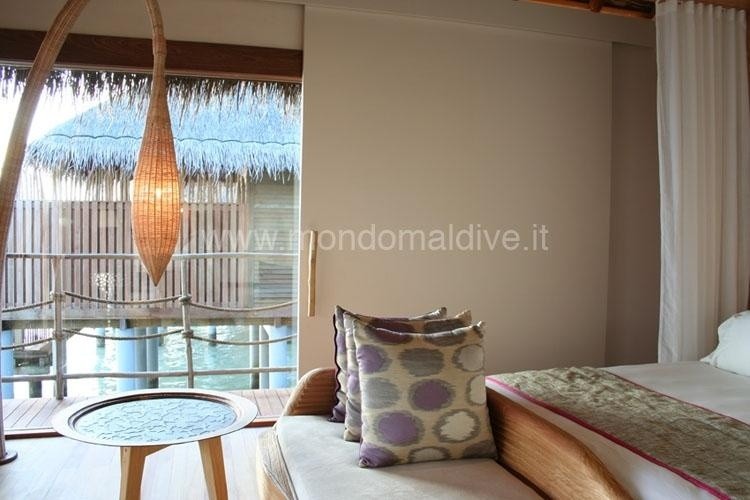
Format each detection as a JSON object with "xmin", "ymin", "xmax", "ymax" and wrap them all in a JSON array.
[{"xmin": 484, "ymin": 359, "xmax": 750, "ymax": 500}]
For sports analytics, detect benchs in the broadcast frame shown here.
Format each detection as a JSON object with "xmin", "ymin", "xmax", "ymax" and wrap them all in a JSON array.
[{"xmin": 266, "ymin": 364, "xmax": 631, "ymax": 500}]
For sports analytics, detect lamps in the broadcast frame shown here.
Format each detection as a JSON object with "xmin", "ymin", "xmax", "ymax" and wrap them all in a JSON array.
[{"xmin": 0, "ymin": 0, "xmax": 184, "ymax": 286}]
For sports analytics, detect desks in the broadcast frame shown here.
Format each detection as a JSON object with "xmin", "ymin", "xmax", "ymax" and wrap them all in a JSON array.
[{"xmin": 52, "ymin": 386, "xmax": 259, "ymax": 500}]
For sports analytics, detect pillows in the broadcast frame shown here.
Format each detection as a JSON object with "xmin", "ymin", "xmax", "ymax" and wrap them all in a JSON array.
[
  {"xmin": 323, "ymin": 303, "xmax": 491, "ymax": 471},
  {"xmin": 699, "ymin": 308, "xmax": 750, "ymax": 379}
]
